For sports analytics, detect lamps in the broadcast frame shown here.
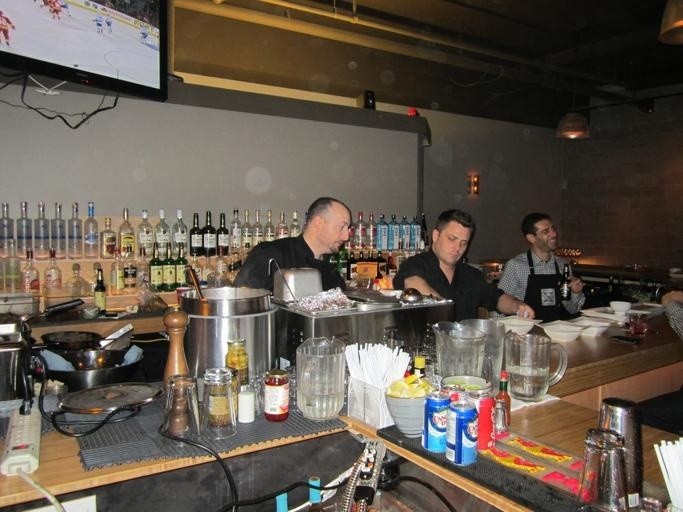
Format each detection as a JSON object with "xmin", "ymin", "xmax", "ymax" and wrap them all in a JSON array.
[
  {"xmin": 657, "ymin": 0, "xmax": 683, "ymax": 46},
  {"xmin": 556, "ymin": 93, "xmax": 591, "ymax": 139},
  {"xmin": 467, "ymin": 174, "xmax": 479, "ymax": 196}
]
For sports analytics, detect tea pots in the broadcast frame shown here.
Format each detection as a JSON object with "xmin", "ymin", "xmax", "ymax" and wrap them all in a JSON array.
[
  {"xmin": 293, "ymin": 335, "xmax": 346, "ymax": 420},
  {"xmin": 428, "ymin": 315, "xmax": 570, "ymax": 404}
]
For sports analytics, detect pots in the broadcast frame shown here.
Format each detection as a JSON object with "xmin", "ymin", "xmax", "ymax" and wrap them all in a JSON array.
[
  {"xmin": 41, "ymin": 330, "xmax": 109, "ymax": 351},
  {"xmin": 179, "ymin": 286, "xmax": 279, "ymax": 382}
]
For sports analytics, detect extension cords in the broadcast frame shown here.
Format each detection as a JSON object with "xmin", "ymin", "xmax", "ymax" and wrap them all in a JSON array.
[{"xmin": 0, "ymin": 406, "xmax": 42, "ymax": 476}]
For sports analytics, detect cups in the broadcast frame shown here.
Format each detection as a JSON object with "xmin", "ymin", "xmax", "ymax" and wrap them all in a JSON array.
[
  {"xmin": 160, "ymin": 375, "xmax": 202, "ymax": 448},
  {"xmin": 574, "ymin": 428, "xmax": 629, "ymax": 512},
  {"xmin": 198, "ymin": 367, "xmax": 240, "ymax": 443}
]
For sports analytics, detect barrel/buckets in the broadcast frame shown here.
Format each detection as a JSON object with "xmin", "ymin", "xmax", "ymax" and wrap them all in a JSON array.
[
  {"xmin": 181, "ymin": 287, "xmax": 274, "ymax": 314},
  {"xmin": 189, "ymin": 312, "xmax": 278, "ymax": 377}
]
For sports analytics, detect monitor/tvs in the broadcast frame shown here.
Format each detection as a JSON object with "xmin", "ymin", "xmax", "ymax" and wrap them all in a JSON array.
[{"xmin": 0, "ymin": 0, "xmax": 169, "ymax": 103}]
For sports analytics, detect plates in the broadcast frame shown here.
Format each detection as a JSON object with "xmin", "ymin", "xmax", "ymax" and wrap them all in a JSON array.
[{"xmin": 579, "ymin": 301, "xmax": 665, "ymax": 325}]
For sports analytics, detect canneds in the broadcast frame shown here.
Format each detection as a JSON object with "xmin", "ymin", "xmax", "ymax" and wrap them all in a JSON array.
[
  {"xmin": 263, "ymin": 370, "xmax": 290, "ymax": 422},
  {"xmin": 422, "ymin": 384, "xmax": 496, "ymax": 467},
  {"xmin": 208, "ymin": 366, "xmax": 238, "ymax": 427}
]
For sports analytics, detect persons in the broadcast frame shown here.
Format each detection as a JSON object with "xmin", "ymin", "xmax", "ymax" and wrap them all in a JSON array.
[
  {"xmin": 1, "ymin": 0, "xmax": 154, "ymax": 48},
  {"xmin": 662, "ymin": 291, "xmax": 683, "ymax": 341},
  {"xmin": 392, "ymin": 209, "xmax": 535, "ymax": 351},
  {"xmin": 232, "ymin": 197, "xmax": 352, "ymax": 365},
  {"xmin": 489, "ymin": 212, "xmax": 586, "ymax": 323}
]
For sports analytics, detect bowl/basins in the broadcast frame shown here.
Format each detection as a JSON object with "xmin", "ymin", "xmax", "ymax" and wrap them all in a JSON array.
[
  {"xmin": 66, "ymin": 349, "xmax": 111, "ymax": 370},
  {"xmin": 383, "ymin": 386, "xmax": 440, "ymax": 440},
  {"xmin": 571, "ymin": 319, "xmax": 611, "ymax": 339},
  {"xmin": 534, "ymin": 322, "xmax": 590, "ymax": 342}
]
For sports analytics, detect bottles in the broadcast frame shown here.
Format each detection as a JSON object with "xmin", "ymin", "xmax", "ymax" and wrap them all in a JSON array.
[
  {"xmin": 263, "ymin": 210, "xmax": 275, "ymax": 241},
  {"xmin": 118, "ymin": 208, "xmax": 136, "ymax": 256},
  {"xmin": 400, "ymin": 216, "xmax": 410, "ymax": 250},
  {"xmin": 67, "ymin": 203, "xmax": 83, "ymax": 258},
  {"xmin": 0, "ymin": 204, "xmax": 16, "ymax": 257},
  {"xmin": 366, "ymin": 212, "xmax": 377, "ymax": 250},
  {"xmin": 83, "ymin": 203, "xmax": 99, "ymax": 257},
  {"xmin": 51, "ymin": 203, "xmax": 67, "ymax": 258},
  {"xmin": 34, "ymin": 203, "xmax": 50, "ymax": 259},
  {"xmin": 0, "ymin": 239, "xmax": 424, "ymax": 314},
  {"xmin": 253, "ymin": 209, "xmax": 263, "ymax": 246},
  {"xmin": 494, "ymin": 371, "xmax": 510, "ymax": 438},
  {"xmin": 344, "ymin": 215, "xmax": 355, "ymax": 249},
  {"xmin": 16, "ymin": 203, "xmax": 33, "ymax": 256},
  {"xmin": 410, "ymin": 216, "xmax": 421, "ymax": 249},
  {"xmin": 202, "ymin": 210, "xmax": 217, "ymax": 257},
  {"xmin": 224, "ymin": 337, "xmax": 250, "ymax": 385},
  {"xmin": 155, "ymin": 208, "xmax": 171, "ymax": 257},
  {"xmin": 306, "ymin": 476, "xmax": 326, "ymax": 512},
  {"xmin": 408, "ymin": 355, "xmax": 427, "ymax": 381},
  {"xmin": 172, "ymin": 209, "xmax": 188, "ymax": 256},
  {"xmin": 276, "ymin": 211, "xmax": 289, "ymax": 239},
  {"xmin": 217, "ymin": 213, "xmax": 230, "ymax": 256},
  {"xmin": 100, "ymin": 218, "xmax": 116, "ymax": 259},
  {"xmin": 388, "ymin": 214, "xmax": 399, "ymax": 250},
  {"xmin": 230, "ymin": 208, "xmax": 242, "ymax": 249},
  {"xmin": 356, "ymin": 212, "xmax": 366, "ymax": 250},
  {"xmin": 290, "ymin": 209, "xmax": 301, "ymax": 237},
  {"xmin": 242, "ymin": 208, "xmax": 252, "ymax": 248},
  {"xmin": 189, "ymin": 212, "xmax": 203, "ymax": 257},
  {"xmin": 559, "ymin": 263, "xmax": 573, "ymax": 302},
  {"xmin": 137, "ymin": 209, "xmax": 154, "ymax": 258},
  {"xmin": 376, "ymin": 213, "xmax": 388, "ymax": 250}
]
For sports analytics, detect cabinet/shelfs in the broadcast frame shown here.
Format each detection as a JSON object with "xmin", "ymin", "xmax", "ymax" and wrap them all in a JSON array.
[{"xmin": 0, "ymin": 249, "xmax": 425, "ymax": 346}]
